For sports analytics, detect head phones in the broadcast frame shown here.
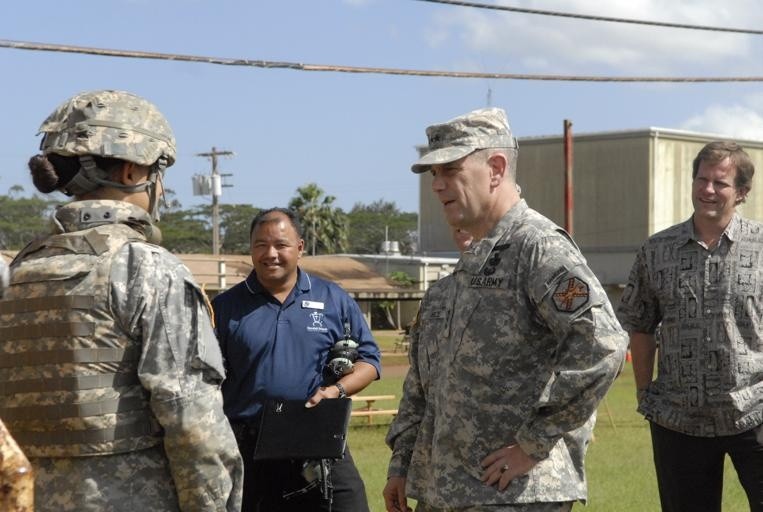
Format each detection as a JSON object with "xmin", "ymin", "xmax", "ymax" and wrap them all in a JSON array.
[{"xmin": 326, "ymin": 316, "xmax": 360, "ymax": 375}]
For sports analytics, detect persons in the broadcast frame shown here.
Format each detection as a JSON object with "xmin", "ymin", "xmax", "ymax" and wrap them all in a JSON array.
[
  {"xmin": 211, "ymin": 208, "xmax": 385, "ymax": 512},
  {"xmin": 618, "ymin": 140, "xmax": 761, "ymax": 511},
  {"xmin": 383, "ymin": 106, "xmax": 633, "ymax": 510},
  {"xmin": 452, "ymin": 176, "xmax": 525, "ymax": 253},
  {"xmin": 1, "ymin": 88, "xmax": 248, "ymax": 512}
]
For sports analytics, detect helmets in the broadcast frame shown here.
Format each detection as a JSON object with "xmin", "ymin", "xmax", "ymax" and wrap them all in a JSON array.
[{"xmin": 35, "ymin": 89, "xmax": 176, "ymax": 169}]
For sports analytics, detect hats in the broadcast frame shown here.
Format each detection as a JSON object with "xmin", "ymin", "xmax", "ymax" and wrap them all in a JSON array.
[{"xmin": 409, "ymin": 106, "xmax": 520, "ymax": 174}]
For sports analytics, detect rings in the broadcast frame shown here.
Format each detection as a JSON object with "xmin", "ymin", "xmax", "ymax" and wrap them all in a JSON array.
[{"xmin": 499, "ymin": 463, "xmax": 510, "ymax": 474}]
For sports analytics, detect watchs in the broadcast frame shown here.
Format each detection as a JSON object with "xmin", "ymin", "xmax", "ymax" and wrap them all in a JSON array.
[{"xmin": 333, "ymin": 383, "xmax": 347, "ymax": 400}]
